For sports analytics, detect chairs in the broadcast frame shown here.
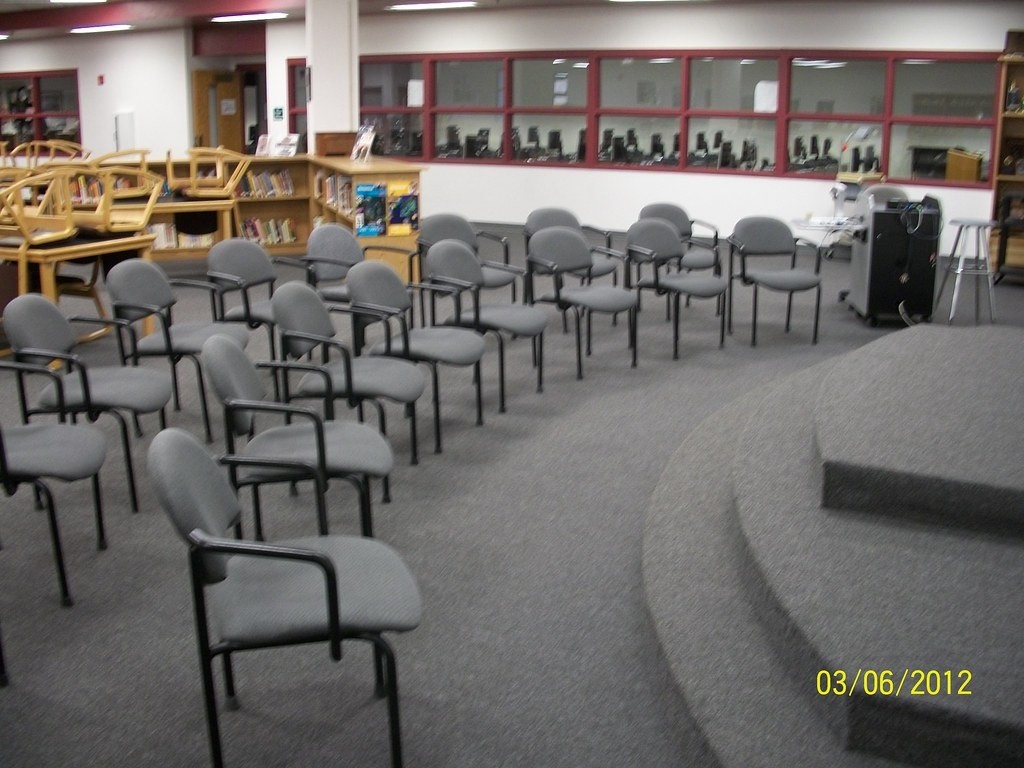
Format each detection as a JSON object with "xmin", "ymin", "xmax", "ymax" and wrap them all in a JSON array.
[
  {"xmin": 0, "ymin": 139, "xmax": 726, "ymax": 688},
  {"xmin": 146, "ymin": 427, "xmax": 422, "ymax": 768},
  {"xmin": 719, "ymin": 214, "xmax": 825, "ymax": 349}
]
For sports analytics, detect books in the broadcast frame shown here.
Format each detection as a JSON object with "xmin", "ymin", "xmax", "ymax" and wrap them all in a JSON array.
[
  {"xmin": 313, "ymin": 169, "xmax": 352, "ymax": 215},
  {"xmin": 70, "ymin": 175, "xmax": 131, "ymax": 205},
  {"xmin": 147, "ymin": 223, "xmax": 219, "ymax": 249},
  {"xmin": 234, "ymin": 170, "xmax": 295, "ymax": 197},
  {"xmin": 240, "ymin": 217, "xmax": 296, "ymax": 245}
]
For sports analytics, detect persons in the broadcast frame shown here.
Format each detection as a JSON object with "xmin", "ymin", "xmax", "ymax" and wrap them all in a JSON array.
[{"xmin": 341, "ymin": 183, "xmax": 351, "ymax": 202}]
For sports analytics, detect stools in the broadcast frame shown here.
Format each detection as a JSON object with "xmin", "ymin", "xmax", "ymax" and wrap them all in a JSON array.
[{"xmin": 926, "ymin": 217, "xmax": 1001, "ymax": 325}]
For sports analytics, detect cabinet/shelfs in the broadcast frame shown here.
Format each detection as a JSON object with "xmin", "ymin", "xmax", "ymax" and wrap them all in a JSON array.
[
  {"xmin": 19, "ymin": 152, "xmax": 423, "ymax": 289},
  {"xmin": 987, "ymin": 53, "xmax": 1024, "ymax": 286}
]
